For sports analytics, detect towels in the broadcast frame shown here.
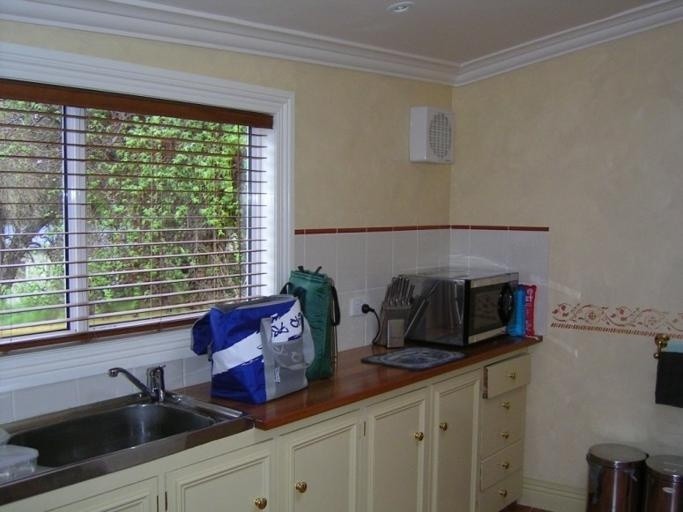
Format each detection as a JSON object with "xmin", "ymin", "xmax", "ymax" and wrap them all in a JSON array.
[{"xmin": 654, "ymin": 352, "xmax": 683, "ymax": 407}]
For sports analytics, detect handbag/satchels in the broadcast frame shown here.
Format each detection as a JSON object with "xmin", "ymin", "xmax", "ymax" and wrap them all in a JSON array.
[
  {"xmin": 279, "ymin": 266, "xmax": 341, "ymax": 379},
  {"xmin": 190, "ymin": 293, "xmax": 317, "ymax": 403}
]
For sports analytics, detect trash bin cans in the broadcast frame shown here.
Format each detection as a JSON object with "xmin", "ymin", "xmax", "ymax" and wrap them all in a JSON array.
[{"xmin": 585, "ymin": 443, "xmax": 683, "ymax": 512}]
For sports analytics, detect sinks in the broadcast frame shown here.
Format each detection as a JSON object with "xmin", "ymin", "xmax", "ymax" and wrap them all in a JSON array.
[{"xmin": 5, "ymin": 392, "xmax": 254, "ymax": 502}]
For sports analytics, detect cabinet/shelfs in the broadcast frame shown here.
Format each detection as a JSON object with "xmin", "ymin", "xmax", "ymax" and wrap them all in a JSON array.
[
  {"xmin": 166, "ymin": 409, "xmax": 365, "ymax": 512},
  {"xmin": 43, "ymin": 475, "xmax": 158, "ymax": 512},
  {"xmin": 481, "ymin": 349, "xmax": 528, "ymax": 511},
  {"xmin": 365, "ymin": 368, "xmax": 480, "ymax": 512}
]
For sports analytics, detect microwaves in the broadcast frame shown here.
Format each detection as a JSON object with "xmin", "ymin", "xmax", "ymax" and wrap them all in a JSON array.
[{"xmin": 402, "ymin": 264, "xmax": 519, "ymax": 348}]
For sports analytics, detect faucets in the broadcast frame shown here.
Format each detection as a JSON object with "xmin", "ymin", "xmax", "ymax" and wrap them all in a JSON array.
[{"xmin": 108, "ymin": 364, "xmax": 165, "ymax": 396}]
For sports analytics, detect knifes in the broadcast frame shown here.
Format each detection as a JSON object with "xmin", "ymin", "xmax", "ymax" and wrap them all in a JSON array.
[
  {"xmin": 382, "ymin": 273, "xmax": 416, "ymax": 307},
  {"xmin": 423, "ymin": 279, "xmax": 441, "ymax": 300}
]
